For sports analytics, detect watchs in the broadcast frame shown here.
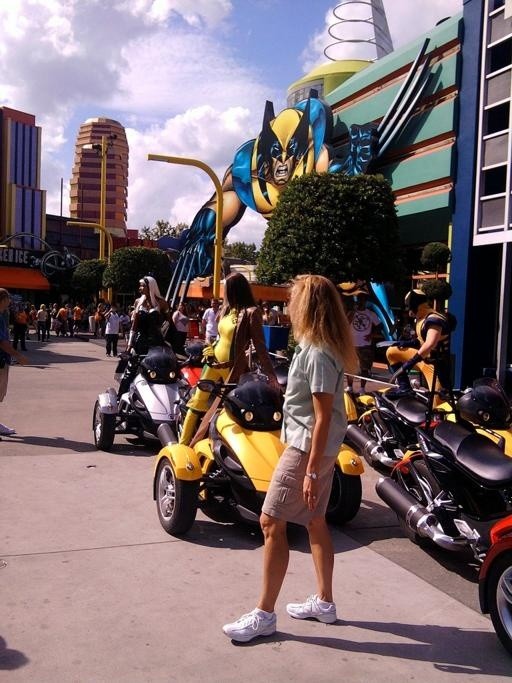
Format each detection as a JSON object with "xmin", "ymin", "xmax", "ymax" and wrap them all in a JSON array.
[{"xmin": 306, "ymin": 472, "xmax": 320, "ymax": 480}]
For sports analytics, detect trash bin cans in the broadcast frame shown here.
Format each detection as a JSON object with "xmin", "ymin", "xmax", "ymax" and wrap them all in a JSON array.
[{"xmin": 88, "ymin": 316, "xmax": 96, "ymax": 332}]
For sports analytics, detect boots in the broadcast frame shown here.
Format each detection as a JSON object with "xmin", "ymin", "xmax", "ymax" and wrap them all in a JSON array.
[{"xmin": 385, "ymin": 362, "xmax": 416, "ymax": 397}]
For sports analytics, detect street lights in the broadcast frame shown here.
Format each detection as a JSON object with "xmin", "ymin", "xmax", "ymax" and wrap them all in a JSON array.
[
  {"xmin": 66, "ymin": 220, "xmax": 112, "ymax": 306},
  {"xmin": 81, "ymin": 136, "xmax": 109, "ymax": 306},
  {"xmin": 229, "ymin": 263, "xmax": 252, "ymax": 281},
  {"xmin": 148, "ymin": 151, "xmax": 223, "ymax": 299}
]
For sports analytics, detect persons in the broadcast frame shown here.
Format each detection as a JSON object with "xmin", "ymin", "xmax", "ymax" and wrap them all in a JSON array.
[
  {"xmin": 10, "ymin": 302, "xmax": 131, "ymax": 357},
  {"xmin": 178, "ymin": 273, "xmax": 286, "ymax": 444},
  {"xmin": 201, "ymin": 299, "xmax": 220, "ymax": 345},
  {"xmin": 118, "ymin": 275, "xmax": 184, "ymax": 403},
  {"xmin": 173, "ymin": 303, "xmax": 189, "ymax": 349},
  {"xmin": 175, "ymin": 302, "xmax": 282, "ymax": 327},
  {"xmin": 386, "ymin": 289, "xmax": 451, "ymax": 422},
  {"xmin": 0, "ymin": 287, "xmax": 29, "ymax": 440},
  {"xmin": 342, "ymin": 293, "xmax": 384, "ymax": 397},
  {"xmin": 221, "ymin": 273, "xmax": 357, "ymax": 644}
]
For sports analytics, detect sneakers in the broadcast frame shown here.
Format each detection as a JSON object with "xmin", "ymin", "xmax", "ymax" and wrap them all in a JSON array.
[
  {"xmin": 0, "ymin": 424, "xmax": 15, "ymax": 434},
  {"xmin": 345, "ymin": 387, "xmax": 352, "ymax": 392},
  {"xmin": 222, "ymin": 609, "xmax": 276, "ymax": 642},
  {"xmin": 359, "ymin": 388, "xmax": 365, "ymax": 395},
  {"xmin": 287, "ymin": 594, "xmax": 336, "ymax": 623}
]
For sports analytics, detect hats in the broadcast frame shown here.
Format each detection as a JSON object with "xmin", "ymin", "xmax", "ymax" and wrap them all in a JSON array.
[{"xmin": 0, "ymin": 288, "xmax": 11, "ymax": 296}]
